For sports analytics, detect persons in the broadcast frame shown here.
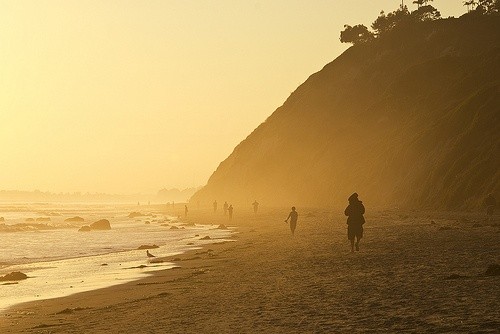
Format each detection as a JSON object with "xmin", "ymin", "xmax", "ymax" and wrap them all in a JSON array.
[
  {"xmin": 227, "ymin": 205, "xmax": 233, "ymax": 218},
  {"xmin": 136, "ymin": 196, "xmax": 218, "ymax": 216},
  {"xmin": 223, "ymin": 201, "xmax": 228, "ymax": 215},
  {"xmin": 344, "ymin": 193, "xmax": 365, "ymax": 251},
  {"xmin": 285, "ymin": 207, "xmax": 298, "ymax": 234},
  {"xmin": 252, "ymin": 200, "xmax": 259, "ymax": 216}
]
